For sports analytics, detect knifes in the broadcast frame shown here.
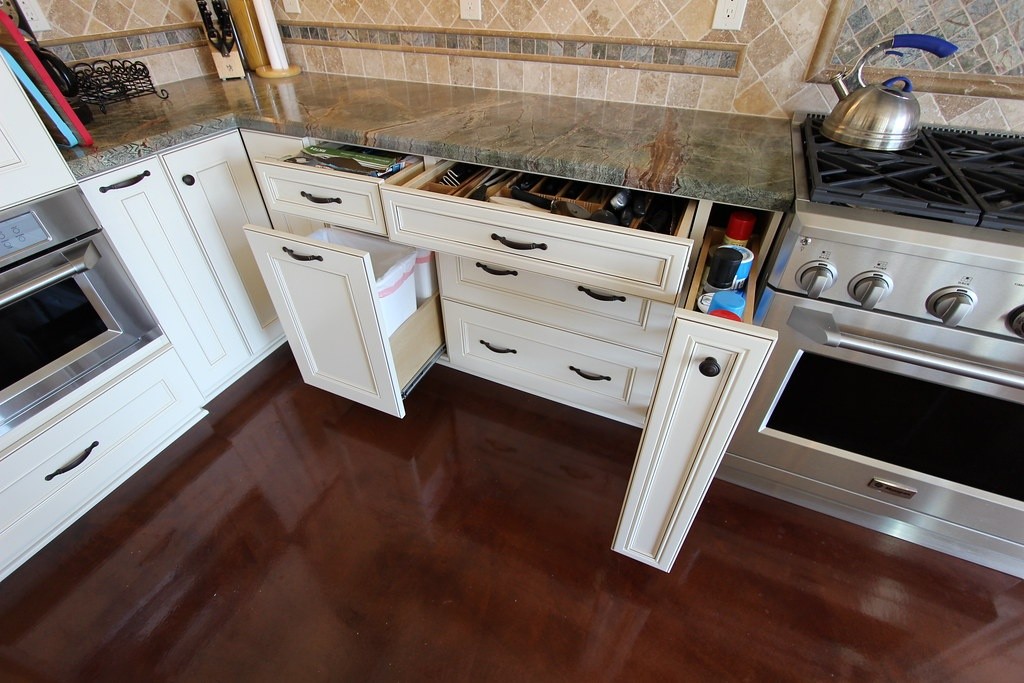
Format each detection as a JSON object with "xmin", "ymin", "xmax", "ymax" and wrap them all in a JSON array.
[{"xmin": 196, "ymin": 0, "xmax": 236, "ymax": 37}]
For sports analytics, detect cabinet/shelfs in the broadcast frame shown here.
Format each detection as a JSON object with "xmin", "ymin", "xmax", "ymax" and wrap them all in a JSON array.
[{"xmin": 0, "ymin": 70, "xmax": 794, "ymax": 584}]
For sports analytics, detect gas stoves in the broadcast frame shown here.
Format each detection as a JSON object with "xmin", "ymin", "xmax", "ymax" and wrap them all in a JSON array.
[{"xmin": 766, "ymin": 109, "xmax": 1022, "ymax": 342}]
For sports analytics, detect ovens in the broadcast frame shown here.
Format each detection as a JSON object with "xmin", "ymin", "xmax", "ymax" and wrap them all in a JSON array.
[
  {"xmin": 712, "ymin": 287, "xmax": 1023, "ymax": 582},
  {"xmin": 0, "ymin": 184, "xmax": 164, "ymax": 443}
]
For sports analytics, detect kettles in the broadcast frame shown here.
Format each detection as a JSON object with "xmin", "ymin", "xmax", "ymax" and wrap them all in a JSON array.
[{"xmin": 820, "ymin": 34, "xmax": 958, "ymax": 151}]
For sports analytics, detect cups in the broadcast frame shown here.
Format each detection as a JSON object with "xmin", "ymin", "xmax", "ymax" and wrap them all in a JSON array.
[{"xmin": 696, "ymin": 213, "xmax": 755, "ymax": 325}]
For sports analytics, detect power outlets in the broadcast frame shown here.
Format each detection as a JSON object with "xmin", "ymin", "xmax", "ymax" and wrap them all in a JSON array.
[
  {"xmin": 460, "ymin": 0, "xmax": 481, "ymax": 21},
  {"xmin": 282, "ymin": 1, "xmax": 300, "ymax": 14},
  {"xmin": 709, "ymin": 0, "xmax": 750, "ymax": 30}
]
